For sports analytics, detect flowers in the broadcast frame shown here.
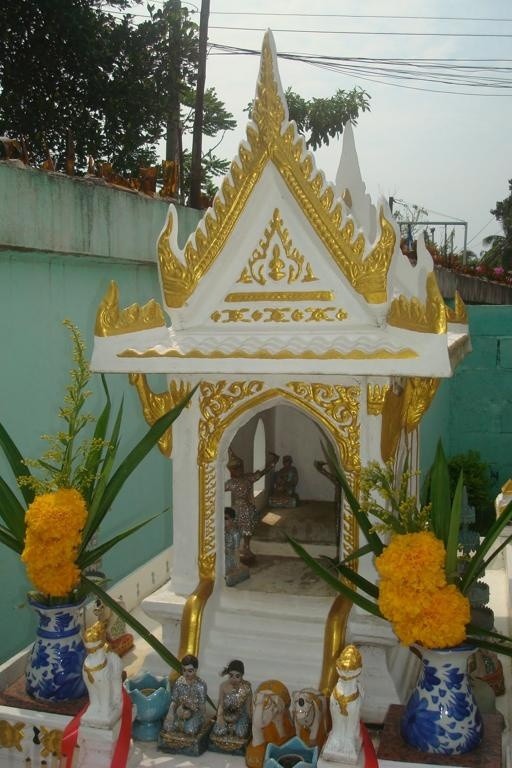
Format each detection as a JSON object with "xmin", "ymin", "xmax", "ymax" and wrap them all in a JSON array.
[
  {"xmin": 1, "ymin": 316, "xmax": 256, "ymax": 717},
  {"xmin": 280, "ymin": 436, "xmax": 512, "ymax": 662}
]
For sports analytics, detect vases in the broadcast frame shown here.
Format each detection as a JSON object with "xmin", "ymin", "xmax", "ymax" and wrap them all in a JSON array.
[
  {"xmin": 25, "ymin": 589, "xmax": 88, "ymax": 705},
  {"xmin": 398, "ymin": 635, "xmax": 483, "ymax": 756}
]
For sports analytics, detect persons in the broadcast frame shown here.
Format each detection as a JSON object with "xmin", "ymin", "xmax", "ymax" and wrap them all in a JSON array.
[
  {"xmin": 272, "ymin": 449, "xmax": 300, "ymax": 504},
  {"xmin": 208, "ymin": 656, "xmax": 253, "ymax": 752},
  {"xmin": 224, "ymin": 507, "xmax": 252, "ymax": 587},
  {"xmin": 224, "ymin": 449, "xmax": 277, "ymax": 562},
  {"xmin": 159, "ymin": 655, "xmax": 212, "ymax": 754}
]
